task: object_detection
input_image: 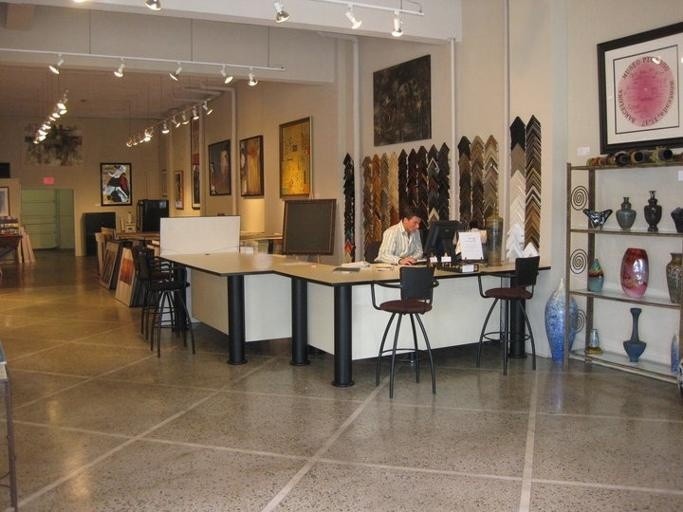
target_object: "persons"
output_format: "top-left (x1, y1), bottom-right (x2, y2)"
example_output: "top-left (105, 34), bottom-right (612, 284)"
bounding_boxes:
top-left (374, 207), bottom-right (423, 266)
top-left (240, 151), bottom-right (247, 196)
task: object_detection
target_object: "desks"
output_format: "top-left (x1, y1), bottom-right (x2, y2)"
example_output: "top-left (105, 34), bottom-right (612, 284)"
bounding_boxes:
top-left (160, 250), bottom-right (281, 365)
top-left (280, 262), bottom-right (550, 392)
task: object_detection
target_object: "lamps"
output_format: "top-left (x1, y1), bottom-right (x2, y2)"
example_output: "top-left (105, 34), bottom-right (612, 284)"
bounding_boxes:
top-left (32, 87), bottom-right (70, 145)
top-left (47, 51), bottom-right (258, 88)
top-left (144, 1), bottom-right (428, 39)
top-left (125, 101), bottom-right (212, 149)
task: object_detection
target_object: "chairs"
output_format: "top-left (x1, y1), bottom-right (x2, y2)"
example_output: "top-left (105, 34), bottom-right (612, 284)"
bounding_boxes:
top-left (368, 265), bottom-right (440, 399)
top-left (131, 246), bottom-right (180, 341)
top-left (478, 256), bottom-right (541, 375)
top-left (137, 250), bottom-right (196, 359)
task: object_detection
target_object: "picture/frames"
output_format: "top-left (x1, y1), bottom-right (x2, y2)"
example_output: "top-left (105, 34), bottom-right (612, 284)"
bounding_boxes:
top-left (278, 114), bottom-right (313, 199)
top-left (207, 139), bottom-right (232, 196)
top-left (0, 184), bottom-right (13, 219)
top-left (239, 135), bottom-right (264, 197)
top-left (96, 239), bottom-right (122, 291)
top-left (595, 18), bottom-right (682, 155)
top-left (114, 246), bottom-right (135, 307)
top-left (160, 115), bottom-right (200, 209)
top-left (100, 162), bottom-right (133, 206)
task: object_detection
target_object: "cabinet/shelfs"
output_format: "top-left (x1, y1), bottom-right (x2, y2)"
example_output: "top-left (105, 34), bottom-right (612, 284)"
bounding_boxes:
top-left (562, 159), bottom-right (683, 388)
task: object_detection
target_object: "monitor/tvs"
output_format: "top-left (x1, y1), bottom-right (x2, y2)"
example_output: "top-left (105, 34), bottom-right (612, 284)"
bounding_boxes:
top-left (423, 220), bottom-right (461, 264)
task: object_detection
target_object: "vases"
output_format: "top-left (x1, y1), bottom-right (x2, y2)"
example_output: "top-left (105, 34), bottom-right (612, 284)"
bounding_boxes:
top-left (665, 249), bottom-right (682, 303)
top-left (616, 246), bottom-right (648, 298)
top-left (669, 203), bottom-right (683, 233)
top-left (670, 328), bottom-right (678, 376)
top-left (542, 280), bottom-right (577, 367)
top-left (621, 304), bottom-right (646, 364)
top-left (614, 198), bottom-right (636, 231)
top-left (586, 257), bottom-right (605, 294)
top-left (641, 189), bottom-right (662, 232)
top-left (589, 329), bottom-right (599, 348)
